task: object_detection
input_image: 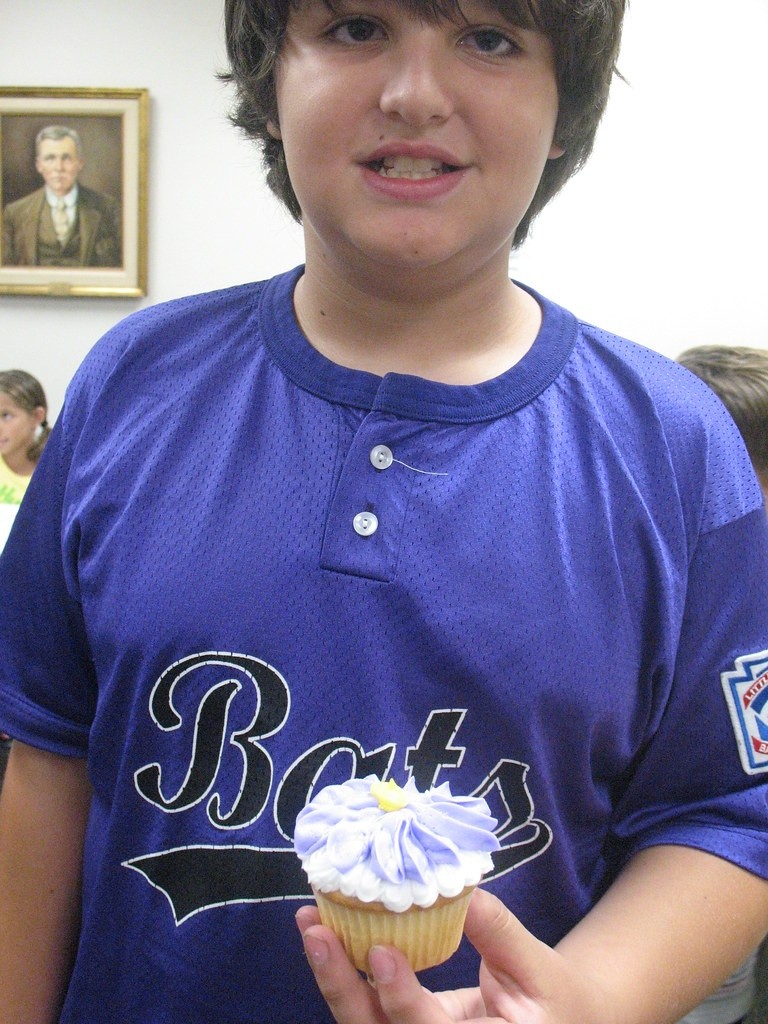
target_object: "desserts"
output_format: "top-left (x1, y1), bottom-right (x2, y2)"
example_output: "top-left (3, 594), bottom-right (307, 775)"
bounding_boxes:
top-left (294, 774), bottom-right (502, 971)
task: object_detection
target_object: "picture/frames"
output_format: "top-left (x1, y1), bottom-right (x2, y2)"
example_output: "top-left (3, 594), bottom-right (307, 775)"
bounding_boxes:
top-left (0, 85), bottom-right (150, 299)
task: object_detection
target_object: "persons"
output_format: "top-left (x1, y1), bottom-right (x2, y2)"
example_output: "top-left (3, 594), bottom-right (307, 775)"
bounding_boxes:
top-left (0, 0), bottom-right (768, 1024)
top-left (674, 346), bottom-right (768, 1024)
top-left (0, 370), bottom-right (52, 504)
